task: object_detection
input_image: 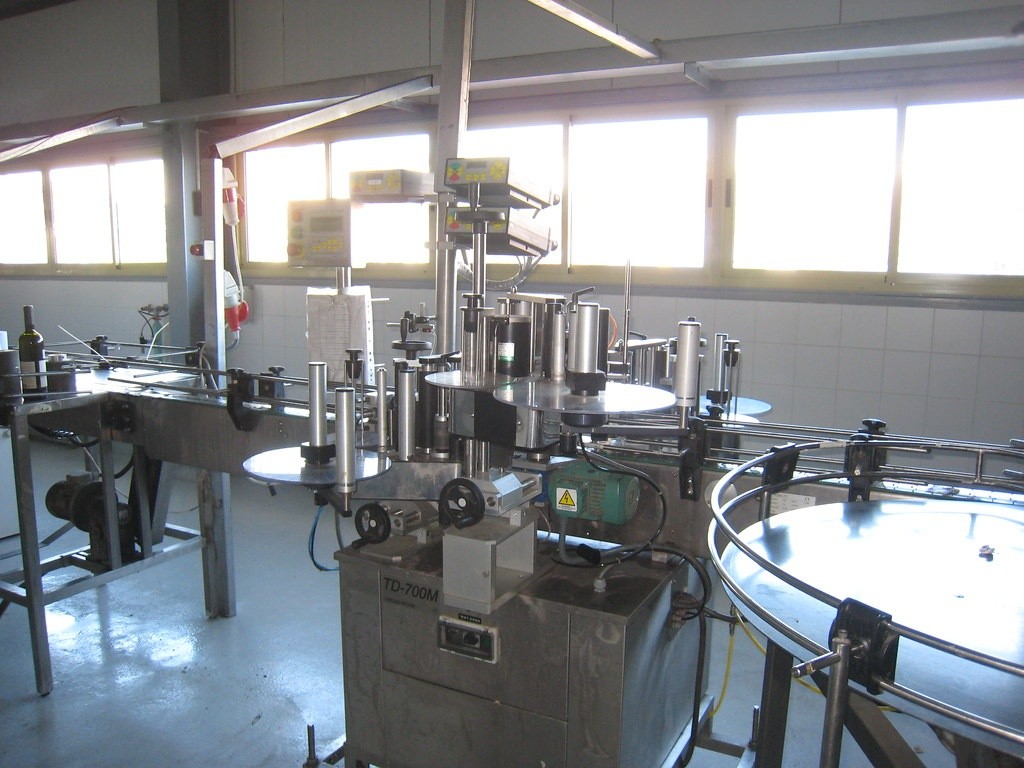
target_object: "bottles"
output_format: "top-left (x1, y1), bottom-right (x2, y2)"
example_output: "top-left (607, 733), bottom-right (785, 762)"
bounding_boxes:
top-left (19, 305), bottom-right (49, 399)
